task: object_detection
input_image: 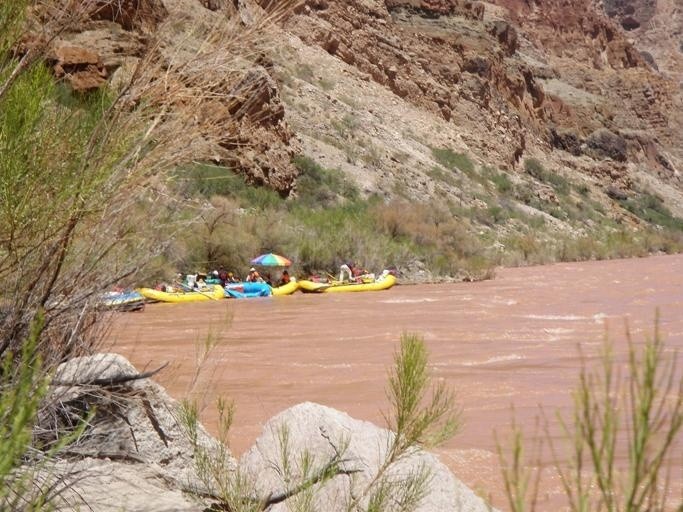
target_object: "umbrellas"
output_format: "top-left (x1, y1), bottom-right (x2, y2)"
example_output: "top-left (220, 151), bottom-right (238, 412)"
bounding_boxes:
top-left (248, 252), bottom-right (292, 268)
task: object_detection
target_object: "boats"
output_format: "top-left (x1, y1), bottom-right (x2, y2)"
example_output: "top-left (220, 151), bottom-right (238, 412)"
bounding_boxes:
top-left (139, 276), bottom-right (297, 304)
top-left (299, 272), bottom-right (396, 293)
top-left (50, 293), bottom-right (144, 312)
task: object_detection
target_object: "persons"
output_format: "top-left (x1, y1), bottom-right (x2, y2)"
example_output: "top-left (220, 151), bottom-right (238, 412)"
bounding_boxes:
top-left (110, 261), bottom-right (402, 297)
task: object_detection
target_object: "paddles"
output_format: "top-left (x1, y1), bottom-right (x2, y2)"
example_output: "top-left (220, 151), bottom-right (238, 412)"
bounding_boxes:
top-left (314, 275), bottom-right (360, 291)
